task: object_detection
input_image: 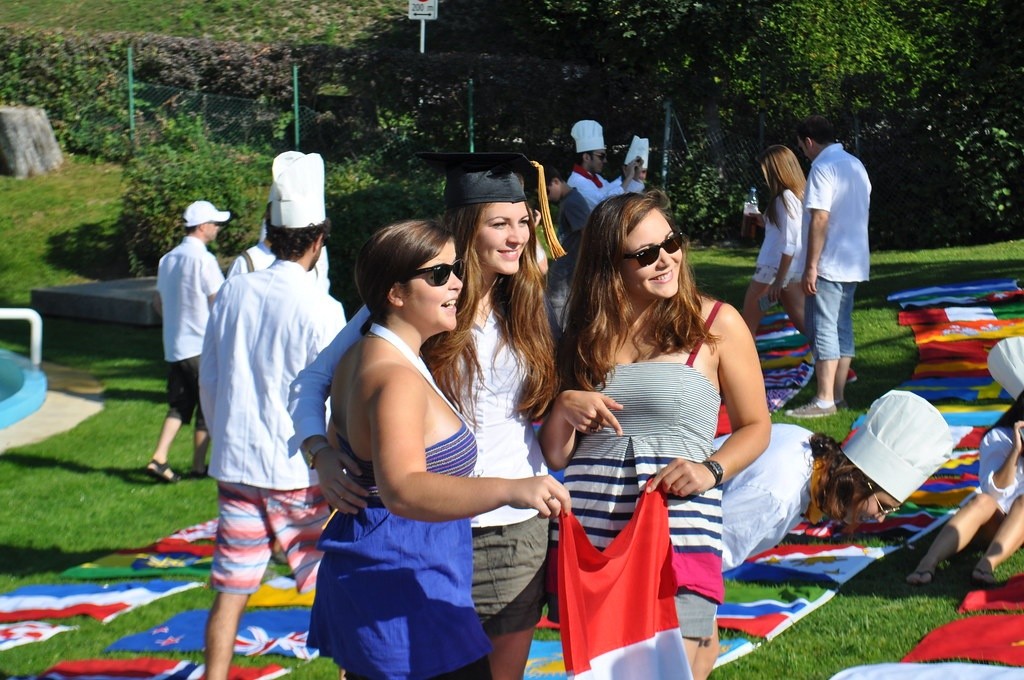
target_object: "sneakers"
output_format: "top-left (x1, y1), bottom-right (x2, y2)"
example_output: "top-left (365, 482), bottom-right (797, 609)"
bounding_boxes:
top-left (785, 400), bottom-right (837, 417)
top-left (836, 401), bottom-right (849, 410)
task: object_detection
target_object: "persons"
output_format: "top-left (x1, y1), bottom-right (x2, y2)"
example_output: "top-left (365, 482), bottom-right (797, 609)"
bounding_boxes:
top-left (784, 115), bottom-right (871, 418)
top-left (710, 389), bottom-right (954, 571)
top-left (286, 153), bottom-right (562, 680)
top-left (906, 337), bottom-right (1024, 582)
top-left (542, 159), bottom-right (591, 334)
top-left (566, 120), bottom-right (644, 211)
top-left (741, 144), bottom-right (857, 383)
top-left (302, 220), bottom-right (572, 680)
top-left (536, 191), bottom-right (772, 680)
top-left (199, 151), bottom-right (347, 680)
top-left (146, 201), bottom-right (231, 481)
top-left (611, 135), bottom-right (649, 185)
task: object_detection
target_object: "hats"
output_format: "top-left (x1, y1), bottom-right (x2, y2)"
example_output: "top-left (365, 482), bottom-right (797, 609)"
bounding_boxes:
top-left (625, 135), bottom-right (649, 169)
top-left (840, 390), bottom-right (953, 503)
top-left (987, 336), bottom-right (1024, 402)
top-left (271, 151), bottom-right (326, 227)
top-left (415, 152), bottom-right (569, 260)
top-left (570, 120), bottom-right (607, 152)
top-left (183, 201), bottom-right (231, 227)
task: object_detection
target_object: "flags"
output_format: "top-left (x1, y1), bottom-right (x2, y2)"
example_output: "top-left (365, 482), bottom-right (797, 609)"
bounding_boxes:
top-left (558, 478), bottom-right (696, 680)
top-left (0, 282), bottom-right (1024, 680)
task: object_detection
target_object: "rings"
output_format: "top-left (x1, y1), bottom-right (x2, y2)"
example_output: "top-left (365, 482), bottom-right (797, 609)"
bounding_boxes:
top-left (545, 496), bottom-right (555, 503)
top-left (591, 423), bottom-right (599, 432)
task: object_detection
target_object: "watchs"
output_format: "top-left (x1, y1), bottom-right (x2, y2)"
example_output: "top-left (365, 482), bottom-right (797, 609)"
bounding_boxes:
top-left (704, 461), bottom-right (723, 485)
top-left (302, 441), bottom-right (329, 469)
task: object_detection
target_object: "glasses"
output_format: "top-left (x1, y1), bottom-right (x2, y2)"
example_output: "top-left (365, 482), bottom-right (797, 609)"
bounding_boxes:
top-left (399, 259), bottom-right (464, 286)
top-left (624, 232), bottom-right (683, 267)
top-left (866, 479), bottom-right (900, 519)
top-left (589, 151), bottom-right (606, 161)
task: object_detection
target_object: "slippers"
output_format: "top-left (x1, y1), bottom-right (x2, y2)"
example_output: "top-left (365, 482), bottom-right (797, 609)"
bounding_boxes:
top-left (973, 569), bottom-right (996, 589)
top-left (906, 570), bottom-right (934, 587)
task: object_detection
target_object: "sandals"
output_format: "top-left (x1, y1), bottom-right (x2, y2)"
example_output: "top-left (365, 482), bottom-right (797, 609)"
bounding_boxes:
top-left (147, 459), bottom-right (182, 482)
top-left (190, 466), bottom-right (208, 480)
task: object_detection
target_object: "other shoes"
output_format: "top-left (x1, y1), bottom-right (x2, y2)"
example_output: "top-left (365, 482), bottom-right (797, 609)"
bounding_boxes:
top-left (847, 369), bottom-right (857, 383)
